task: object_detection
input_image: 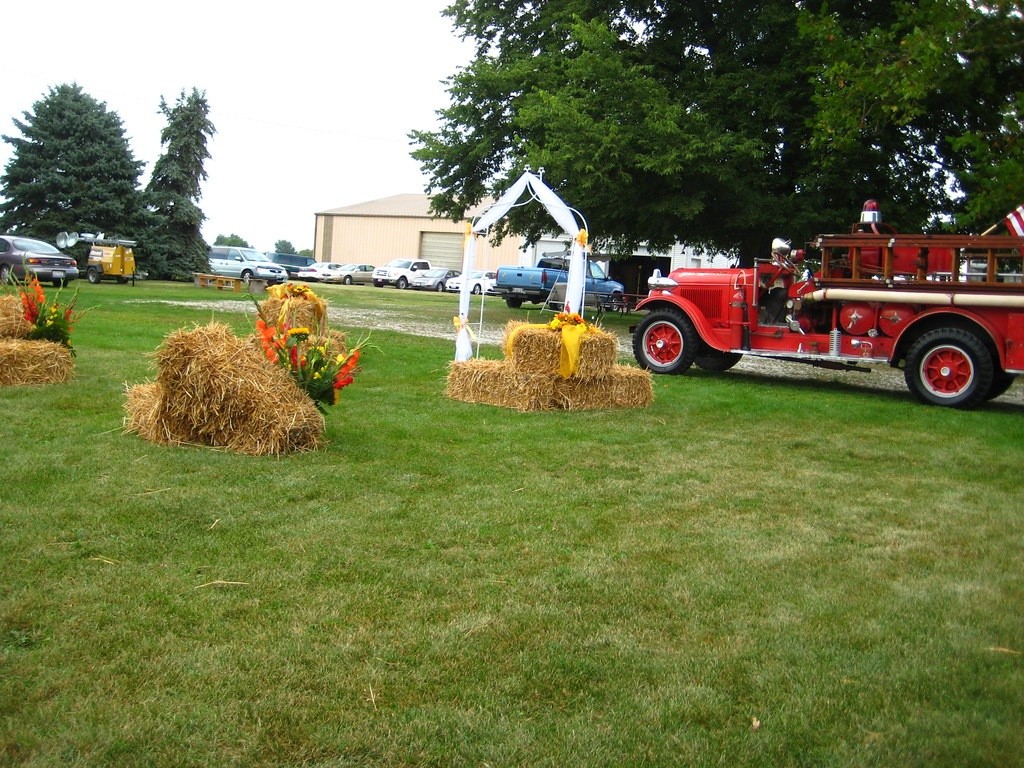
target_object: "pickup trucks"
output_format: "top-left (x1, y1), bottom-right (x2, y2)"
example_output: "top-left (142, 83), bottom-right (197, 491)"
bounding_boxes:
top-left (493, 258), bottom-right (624, 312)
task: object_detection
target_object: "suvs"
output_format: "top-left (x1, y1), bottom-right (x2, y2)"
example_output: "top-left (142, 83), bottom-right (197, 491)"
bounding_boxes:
top-left (372, 259), bottom-right (431, 289)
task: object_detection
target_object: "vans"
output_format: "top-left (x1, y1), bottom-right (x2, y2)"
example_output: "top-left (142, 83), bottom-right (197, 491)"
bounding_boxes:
top-left (273, 253), bottom-right (317, 280)
top-left (209, 246), bottom-right (288, 283)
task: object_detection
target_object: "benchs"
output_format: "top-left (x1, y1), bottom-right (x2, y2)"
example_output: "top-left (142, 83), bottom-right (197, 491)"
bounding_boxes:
top-left (194, 272), bottom-right (268, 294)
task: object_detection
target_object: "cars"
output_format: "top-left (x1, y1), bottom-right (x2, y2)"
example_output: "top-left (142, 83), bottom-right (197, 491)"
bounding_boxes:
top-left (320, 264), bottom-right (376, 285)
top-left (445, 271), bottom-right (497, 295)
top-left (0, 236), bottom-right (79, 288)
top-left (412, 268), bottom-right (461, 293)
top-left (297, 263), bottom-right (342, 282)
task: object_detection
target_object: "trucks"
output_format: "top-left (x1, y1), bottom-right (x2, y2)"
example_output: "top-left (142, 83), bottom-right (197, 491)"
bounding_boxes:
top-left (629, 199), bottom-right (1024, 407)
